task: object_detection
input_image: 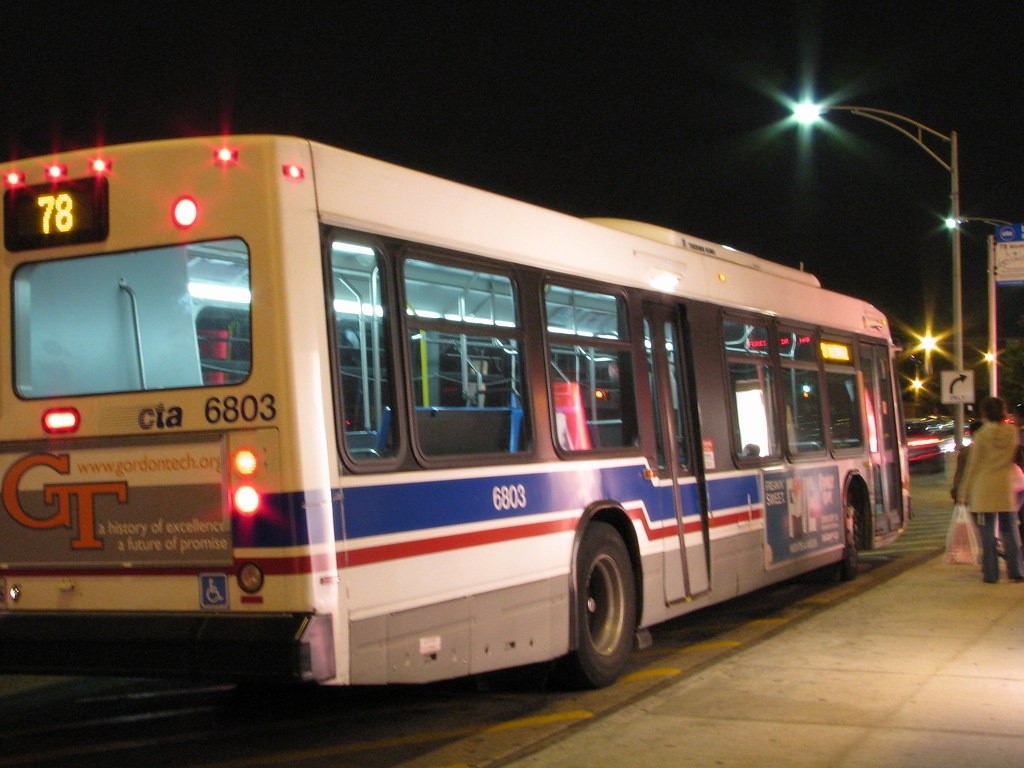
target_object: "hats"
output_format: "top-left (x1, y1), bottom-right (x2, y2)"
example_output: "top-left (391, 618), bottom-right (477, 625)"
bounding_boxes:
top-left (744, 444), bottom-right (760, 453)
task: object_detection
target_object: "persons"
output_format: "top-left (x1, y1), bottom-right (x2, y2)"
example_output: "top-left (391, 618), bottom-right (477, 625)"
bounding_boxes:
top-left (956, 395), bottom-right (1024, 583)
top-left (951, 406), bottom-right (1024, 573)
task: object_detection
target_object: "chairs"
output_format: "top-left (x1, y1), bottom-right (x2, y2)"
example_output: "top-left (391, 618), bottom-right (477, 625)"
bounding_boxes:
top-left (549, 382), bottom-right (593, 452)
top-left (787, 442), bottom-right (820, 454)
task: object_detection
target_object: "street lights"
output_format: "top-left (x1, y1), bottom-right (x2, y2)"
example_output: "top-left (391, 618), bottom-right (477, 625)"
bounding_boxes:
top-left (794, 99), bottom-right (964, 452)
top-left (941, 213), bottom-right (1012, 402)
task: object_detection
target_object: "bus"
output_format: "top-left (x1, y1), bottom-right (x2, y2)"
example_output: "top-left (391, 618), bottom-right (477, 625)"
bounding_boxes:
top-left (0, 133), bottom-right (912, 689)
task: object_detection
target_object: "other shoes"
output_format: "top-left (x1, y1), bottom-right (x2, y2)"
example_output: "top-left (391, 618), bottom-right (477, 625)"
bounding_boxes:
top-left (1015, 576), bottom-right (1024, 581)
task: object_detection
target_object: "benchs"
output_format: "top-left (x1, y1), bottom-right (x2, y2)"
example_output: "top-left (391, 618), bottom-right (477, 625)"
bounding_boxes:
top-left (347, 406), bottom-right (528, 462)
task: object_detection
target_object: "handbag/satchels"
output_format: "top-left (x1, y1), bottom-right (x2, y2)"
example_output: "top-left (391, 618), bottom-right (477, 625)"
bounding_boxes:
top-left (943, 505), bottom-right (979, 565)
